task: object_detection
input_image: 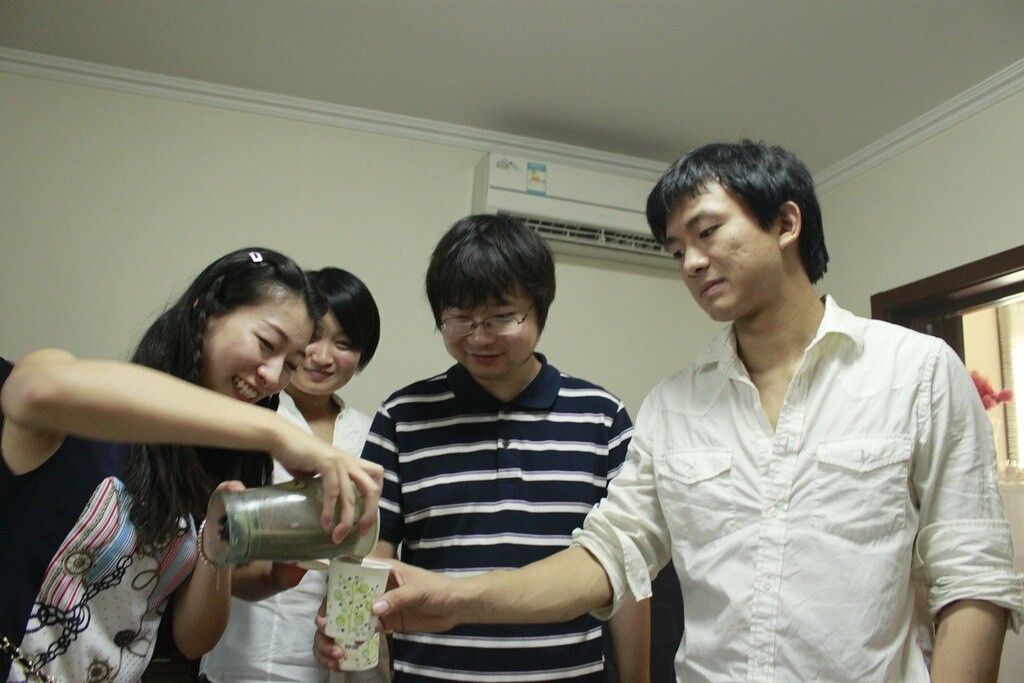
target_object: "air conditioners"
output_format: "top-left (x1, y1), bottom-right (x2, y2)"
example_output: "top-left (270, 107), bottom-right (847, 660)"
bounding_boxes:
top-left (469, 152), bottom-right (681, 273)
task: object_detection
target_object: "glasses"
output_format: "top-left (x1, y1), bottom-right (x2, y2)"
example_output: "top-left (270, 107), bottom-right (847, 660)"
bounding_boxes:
top-left (434, 301), bottom-right (536, 337)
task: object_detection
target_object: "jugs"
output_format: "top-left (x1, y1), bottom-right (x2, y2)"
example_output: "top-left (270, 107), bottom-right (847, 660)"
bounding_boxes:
top-left (207, 476), bottom-right (380, 563)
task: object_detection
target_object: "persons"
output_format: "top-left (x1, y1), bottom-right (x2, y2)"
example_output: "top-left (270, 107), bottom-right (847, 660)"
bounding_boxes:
top-left (0, 247), bottom-right (384, 683)
top-left (315, 214), bottom-right (652, 683)
top-left (321, 141), bottom-right (1022, 683)
top-left (198, 265), bottom-right (381, 683)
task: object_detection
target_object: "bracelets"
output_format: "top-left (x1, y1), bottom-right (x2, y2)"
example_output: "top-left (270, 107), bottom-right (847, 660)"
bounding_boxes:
top-left (198, 517), bottom-right (231, 572)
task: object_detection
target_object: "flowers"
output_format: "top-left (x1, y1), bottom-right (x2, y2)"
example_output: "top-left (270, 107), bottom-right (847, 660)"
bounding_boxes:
top-left (968, 371), bottom-right (1013, 409)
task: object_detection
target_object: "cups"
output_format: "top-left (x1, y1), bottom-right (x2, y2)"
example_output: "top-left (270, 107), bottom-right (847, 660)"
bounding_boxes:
top-left (325, 558), bottom-right (392, 641)
top-left (332, 632), bottom-right (380, 672)
